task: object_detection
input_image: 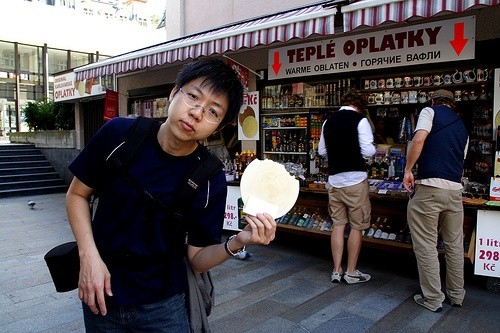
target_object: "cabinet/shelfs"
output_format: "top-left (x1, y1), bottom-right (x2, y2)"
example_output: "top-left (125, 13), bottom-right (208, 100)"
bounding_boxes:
top-left (258, 64), bottom-right (491, 254)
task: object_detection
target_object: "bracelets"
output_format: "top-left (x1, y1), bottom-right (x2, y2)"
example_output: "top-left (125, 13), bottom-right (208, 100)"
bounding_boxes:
top-left (225, 235), bottom-right (245, 257)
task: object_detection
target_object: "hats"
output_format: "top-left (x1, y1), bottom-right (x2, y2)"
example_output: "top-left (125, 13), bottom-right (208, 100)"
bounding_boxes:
top-left (425, 89), bottom-right (454, 107)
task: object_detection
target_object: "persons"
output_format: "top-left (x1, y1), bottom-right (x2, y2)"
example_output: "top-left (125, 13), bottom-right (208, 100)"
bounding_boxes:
top-left (403, 89), bottom-right (469, 312)
top-left (318, 89), bottom-right (377, 285)
top-left (65, 58), bottom-right (277, 333)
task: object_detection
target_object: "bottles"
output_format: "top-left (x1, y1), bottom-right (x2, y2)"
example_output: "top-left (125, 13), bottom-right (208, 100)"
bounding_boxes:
top-left (370, 157), bottom-right (402, 177)
top-left (262, 78), bottom-right (351, 183)
top-left (235, 150), bottom-right (256, 173)
top-left (274, 205), bottom-right (470, 253)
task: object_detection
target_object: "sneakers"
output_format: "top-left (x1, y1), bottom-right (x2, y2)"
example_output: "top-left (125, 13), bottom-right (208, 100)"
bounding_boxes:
top-left (343, 270), bottom-right (372, 284)
top-left (451, 301), bottom-right (462, 307)
top-left (414, 294), bottom-right (443, 312)
top-left (331, 272), bottom-right (344, 282)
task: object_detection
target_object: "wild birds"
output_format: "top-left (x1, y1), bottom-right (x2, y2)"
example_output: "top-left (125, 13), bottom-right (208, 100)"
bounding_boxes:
top-left (27, 200), bottom-right (36, 209)
top-left (238, 250), bottom-right (254, 260)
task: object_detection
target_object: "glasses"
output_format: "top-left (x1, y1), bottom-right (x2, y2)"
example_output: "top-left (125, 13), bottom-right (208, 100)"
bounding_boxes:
top-left (180, 88), bottom-right (223, 124)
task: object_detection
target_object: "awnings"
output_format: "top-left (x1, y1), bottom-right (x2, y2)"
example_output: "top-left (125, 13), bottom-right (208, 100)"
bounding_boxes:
top-left (340, 0), bottom-right (500, 32)
top-left (74, 0), bottom-right (337, 82)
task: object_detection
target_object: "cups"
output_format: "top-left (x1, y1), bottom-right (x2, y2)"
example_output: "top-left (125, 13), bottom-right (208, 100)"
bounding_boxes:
top-left (365, 69), bottom-right (488, 105)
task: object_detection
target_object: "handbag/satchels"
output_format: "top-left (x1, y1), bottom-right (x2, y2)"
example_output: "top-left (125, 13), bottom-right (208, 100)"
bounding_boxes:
top-left (44, 242), bottom-right (81, 293)
top-left (196, 270), bottom-right (215, 316)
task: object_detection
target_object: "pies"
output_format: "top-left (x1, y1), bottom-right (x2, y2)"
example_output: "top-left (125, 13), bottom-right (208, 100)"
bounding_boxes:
top-left (240, 158), bottom-right (299, 219)
